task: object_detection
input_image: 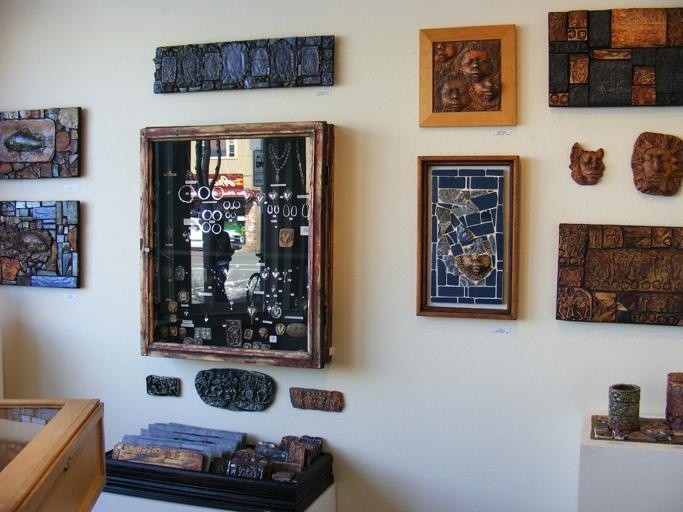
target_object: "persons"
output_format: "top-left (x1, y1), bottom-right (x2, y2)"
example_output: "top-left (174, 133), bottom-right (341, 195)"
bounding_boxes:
top-left (578, 148), bottom-right (604, 185)
top-left (434, 41), bottom-right (499, 113)
top-left (641, 146), bottom-right (673, 186)
top-left (456, 252), bottom-right (494, 280)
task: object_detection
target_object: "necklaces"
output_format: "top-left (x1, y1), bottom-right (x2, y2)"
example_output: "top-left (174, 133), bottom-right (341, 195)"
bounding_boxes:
top-left (295, 135), bottom-right (305, 184)
top-left (266, 143), bottom-right (292, 184)
top-left (191, 257), bottom-right (307, 325)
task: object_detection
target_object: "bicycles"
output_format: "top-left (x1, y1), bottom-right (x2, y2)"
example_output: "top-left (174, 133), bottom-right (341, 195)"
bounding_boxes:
top-left (245, 252), bottom-right (265, 305)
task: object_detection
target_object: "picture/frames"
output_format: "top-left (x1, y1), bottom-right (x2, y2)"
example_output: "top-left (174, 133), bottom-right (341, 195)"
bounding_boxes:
top-left (418, 24), bottom-right (518, 128)
top-left (140, 121), bottom-right (335, 369)
top-left (415, 155), bottom-right (520, 320)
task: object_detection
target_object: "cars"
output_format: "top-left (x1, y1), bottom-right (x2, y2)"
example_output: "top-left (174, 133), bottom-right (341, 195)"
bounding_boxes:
top-left (184, 217), bottom-right (242, 253)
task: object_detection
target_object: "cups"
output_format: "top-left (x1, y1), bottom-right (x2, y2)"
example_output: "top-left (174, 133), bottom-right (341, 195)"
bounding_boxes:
top-left (667, 372), bottom-right (682, 432)
